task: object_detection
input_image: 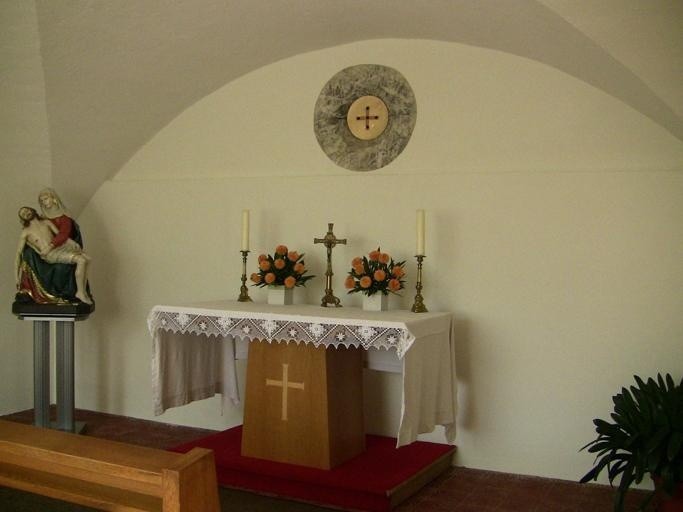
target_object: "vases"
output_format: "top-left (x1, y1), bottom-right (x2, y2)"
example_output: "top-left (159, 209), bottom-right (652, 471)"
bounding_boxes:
top-left (362, 290), bottom-right (388, 311)
top-left (268, 289), bottom-right (293, 305)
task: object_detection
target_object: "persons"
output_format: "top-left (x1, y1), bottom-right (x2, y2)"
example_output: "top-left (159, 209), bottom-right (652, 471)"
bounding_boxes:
top-left (14, 207), bottom-right (93, 306)
top-left (15, 188), bottom-right (90, 305)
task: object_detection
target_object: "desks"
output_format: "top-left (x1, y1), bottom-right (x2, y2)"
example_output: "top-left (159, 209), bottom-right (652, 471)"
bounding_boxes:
top-left (148, 300), bottom-right (457, 511)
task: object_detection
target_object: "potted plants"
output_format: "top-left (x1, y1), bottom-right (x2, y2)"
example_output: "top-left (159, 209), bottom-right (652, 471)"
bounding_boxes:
top-left (580, 372), bottom-right (683, 512)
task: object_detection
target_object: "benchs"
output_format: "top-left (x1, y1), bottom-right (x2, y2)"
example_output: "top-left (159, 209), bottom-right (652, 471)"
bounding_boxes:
top-left (0, 419), bottom-right (221, 512)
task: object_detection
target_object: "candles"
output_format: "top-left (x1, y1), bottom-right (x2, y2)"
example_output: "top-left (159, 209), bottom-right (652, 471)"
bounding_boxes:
top-left (242, 210), bottom-right (249, 251)
top-left (417, 209), bottom-right (425, 255)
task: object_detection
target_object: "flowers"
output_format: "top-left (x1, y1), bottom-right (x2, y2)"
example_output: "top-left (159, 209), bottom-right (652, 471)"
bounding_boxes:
top-left (249, 246), bottom-right (315, 289)
top-left (345, 246), bottom-right (406, 297)
